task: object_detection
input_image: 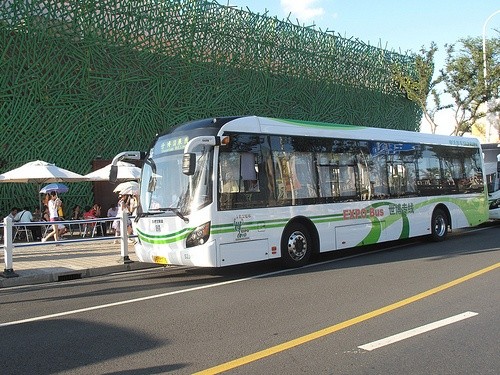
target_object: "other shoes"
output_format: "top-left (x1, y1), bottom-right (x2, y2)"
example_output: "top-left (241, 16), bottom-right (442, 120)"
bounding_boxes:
top-left (41, 238), bottom-right (46, 242)
top-left (128, 239), bottom-right (133, 243)
top-left (114, 239), bottom-right (119, 244)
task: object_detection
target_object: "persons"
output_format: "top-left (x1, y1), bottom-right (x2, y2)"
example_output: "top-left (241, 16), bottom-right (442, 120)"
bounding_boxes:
top-left (83, 191), bottom-right (139, 244)
top-left (33, 207), bottom-right (46, 222)
top-left (58, 204), bottom-right (64, 217)
top-left (13, 207), bottom-right (38, 242)
top-left (70, 205), bottom-right (86, 229)
top-left (42, 191), bottom-right (65, 247)
top-left (41, 193), bottom-right (51, 221)
top-left (6, 207), bottom-right (18, 235)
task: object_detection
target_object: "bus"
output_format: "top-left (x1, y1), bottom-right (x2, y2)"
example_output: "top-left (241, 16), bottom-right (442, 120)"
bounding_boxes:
top-left (109, 116), bottom-right (500, 268)
top-left (480, 144), bottom-right (499, 217)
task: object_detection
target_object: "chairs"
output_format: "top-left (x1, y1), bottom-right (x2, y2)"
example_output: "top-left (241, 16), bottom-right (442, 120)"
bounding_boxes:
top-left (12, 224), bottom-right (33, 243)
top-left (83, 216), bottom-right (104, 237)
top-left (69, 223), bottom-right (84, 237)
top-left (42, 218), bottom-right (60, 242)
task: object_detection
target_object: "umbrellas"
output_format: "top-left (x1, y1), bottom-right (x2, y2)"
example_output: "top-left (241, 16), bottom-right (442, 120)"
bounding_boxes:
top-left (85, 158), bottom-right (164, 182)
top-left (112, 182), bottom-right (141, 192)
top-left (0, 159), bottom-right (85, 213)
top-left (38, 183), bottom-right (70, 194)
top-left (118, 186), bottom-right (142, 197)
